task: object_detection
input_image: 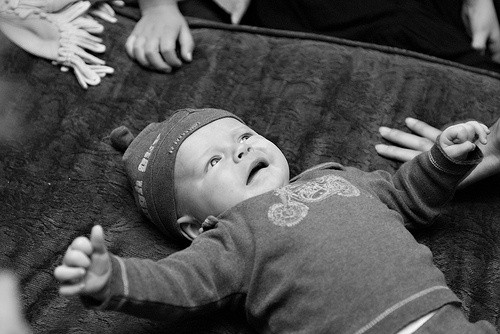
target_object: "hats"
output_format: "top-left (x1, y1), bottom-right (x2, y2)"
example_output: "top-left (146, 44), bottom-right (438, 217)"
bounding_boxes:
top-left (109, 107), bottom-right (245, 242)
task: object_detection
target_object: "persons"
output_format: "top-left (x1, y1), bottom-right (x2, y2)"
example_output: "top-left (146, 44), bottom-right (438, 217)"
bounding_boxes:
top-left (127, 0), bottom-right (500, 72)
top-left (55, 108), bottom-right (497, 334)
top-left (376, 117), bottom-right (500, 184)
